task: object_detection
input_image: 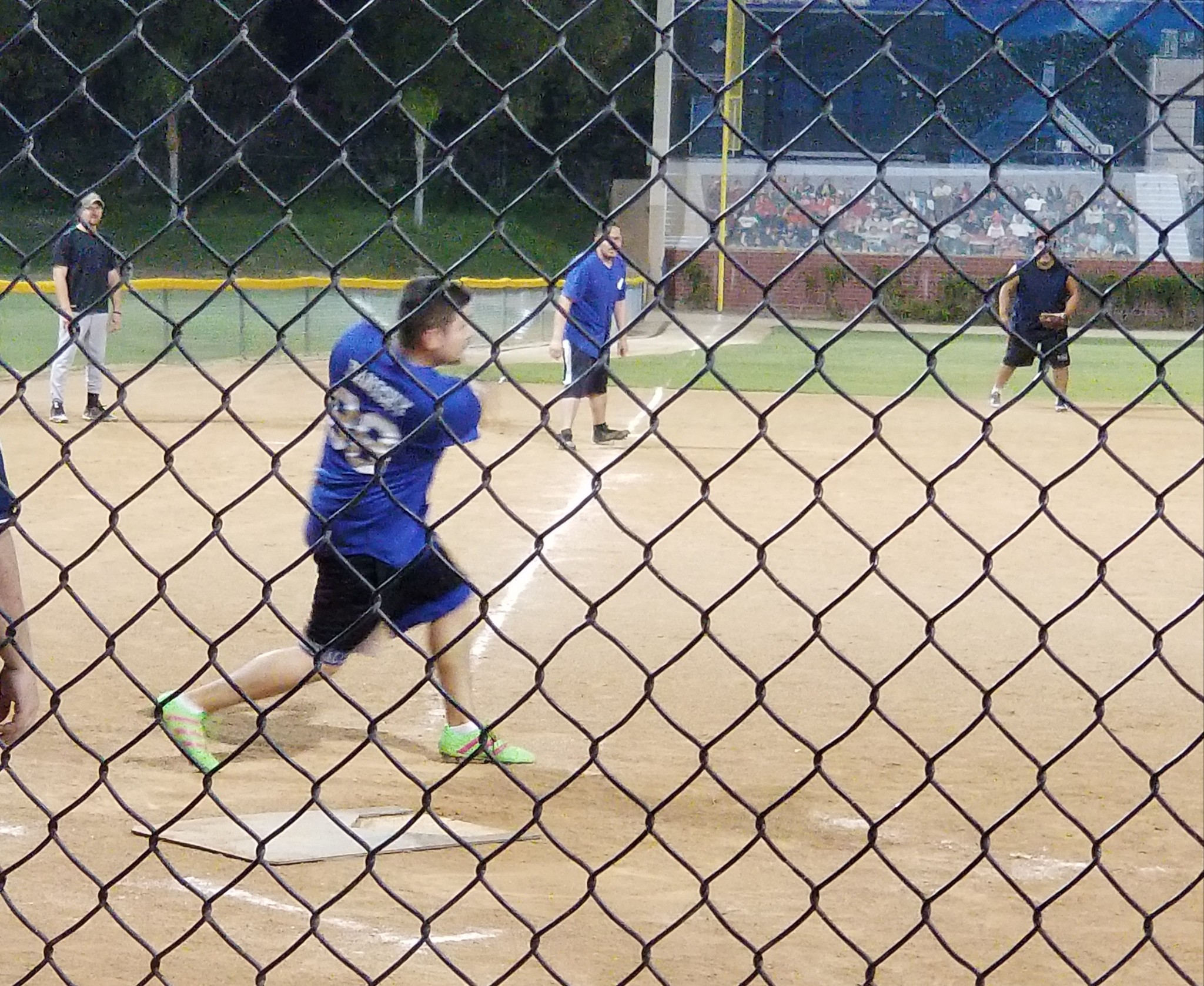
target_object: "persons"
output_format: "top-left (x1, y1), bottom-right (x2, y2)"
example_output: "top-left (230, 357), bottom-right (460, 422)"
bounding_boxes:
top-left (989, 235), bottom-right (1081, 411)
top-left (0, 454), bottom-right (41, 749)
top-left (159, 274), bottom-right (536, 774)
top-left (48, 192), bottom-right (122, 423)
top-left (706, 177), bottom-right (1137, 259)
top-left (550, 221), bottom-right (631, 451)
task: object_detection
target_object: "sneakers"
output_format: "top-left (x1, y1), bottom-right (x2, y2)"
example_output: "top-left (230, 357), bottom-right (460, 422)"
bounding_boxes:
top-left (554, 429), bottom-right (577, 451)
top-left (991, 391), bottom-right (1001, 406)
top-left (1055, 397), bottom-right (1068, 411)
top-left (83, 399), bottom-right (119, 422)
top-left (149, 691), bottom-right (221, 773)
top-left (592, 425), bottom-right (631, 444)
top-left (49, 402), bottom-right (69, 423)
top-left (439, 725), bottom-right (535, 765)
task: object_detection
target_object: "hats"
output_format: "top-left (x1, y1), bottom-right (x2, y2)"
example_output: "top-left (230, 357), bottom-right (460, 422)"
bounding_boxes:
top-left (81, 192), bottom-right (105, 210)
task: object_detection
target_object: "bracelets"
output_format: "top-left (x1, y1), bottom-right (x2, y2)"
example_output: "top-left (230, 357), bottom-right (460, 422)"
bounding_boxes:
top-left (113, 311), bottom-right (121, 314)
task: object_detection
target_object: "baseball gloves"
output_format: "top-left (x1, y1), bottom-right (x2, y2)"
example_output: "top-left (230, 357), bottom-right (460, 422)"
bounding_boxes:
top-left (1039, 313), bottom-right (1069, 331)
